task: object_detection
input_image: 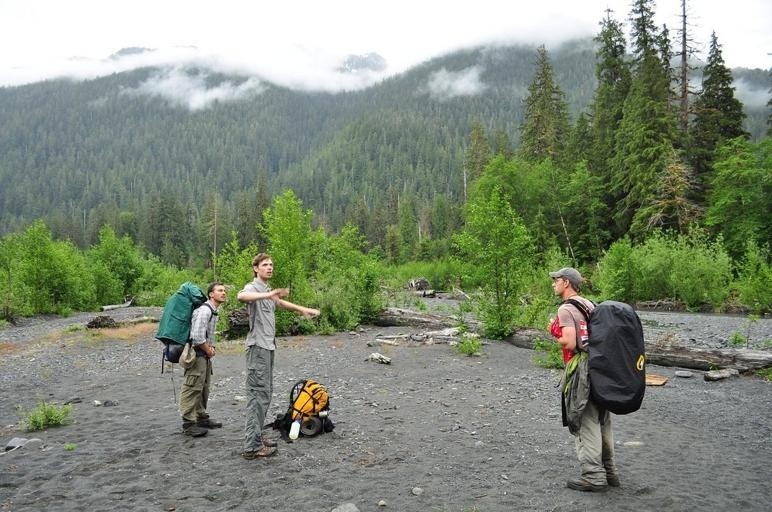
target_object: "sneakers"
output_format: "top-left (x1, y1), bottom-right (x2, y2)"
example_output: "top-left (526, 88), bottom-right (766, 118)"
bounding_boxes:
top-left (199, 420), bottom-right (222, 429)
top-left (262, 441), bottom-right (278, 447)
top-left (245, 445), bottom-right (277, 459)
top-left (184, 425), bottom-right (208, 437)
top-left (566, 475), bottom-right (620, 493)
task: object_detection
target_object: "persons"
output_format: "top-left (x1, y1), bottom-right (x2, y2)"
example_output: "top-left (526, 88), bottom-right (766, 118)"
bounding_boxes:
top-left (548, 267), bottom-right (621, 494)
top-left (178, 283), bottom-right (227, 438)
top-left (236, 253), bottom-right (321, 459)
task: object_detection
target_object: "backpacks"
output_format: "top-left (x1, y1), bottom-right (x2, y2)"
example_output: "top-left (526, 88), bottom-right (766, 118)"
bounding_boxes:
top-left (276, 379), bottom-right (329, 436)
top-left (155, 281), bottom-right (208, 363)
top-left (567, 296), bottom-right (645, 415)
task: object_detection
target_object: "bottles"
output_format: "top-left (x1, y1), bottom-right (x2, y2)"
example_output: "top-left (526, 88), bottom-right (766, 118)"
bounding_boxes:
top-left (289, 420), bottom-right (301, 440)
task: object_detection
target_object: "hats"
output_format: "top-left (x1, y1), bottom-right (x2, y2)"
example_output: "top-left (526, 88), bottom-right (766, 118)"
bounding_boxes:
top-left (549, 267), bottom-right (582, 285)
top-left (179, 344), bottom-right (197, 369)
